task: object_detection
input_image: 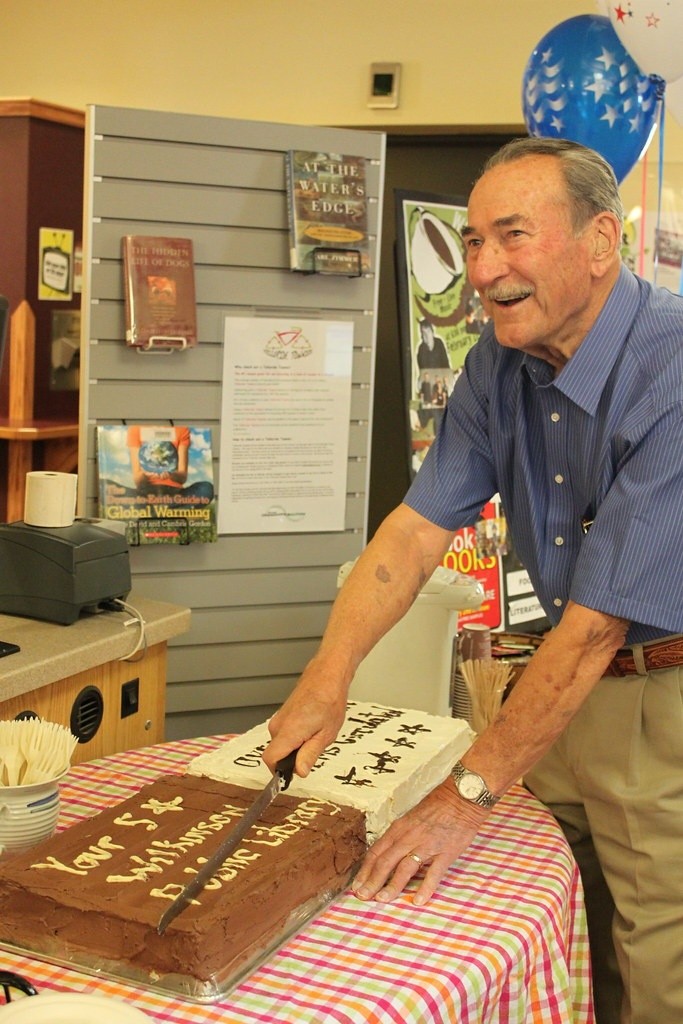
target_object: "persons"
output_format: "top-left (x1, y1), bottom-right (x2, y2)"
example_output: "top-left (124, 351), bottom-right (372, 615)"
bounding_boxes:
top-left (419, 373), bottom-right (449, 410)
top-left (416, 319), bottom-right (451, 370)
top-left (466, 297), bottom-right (487, 333)
top-left (478, 525), bottom-right (504, 555)
top-left (263, 131), bottom-right (683, 1024)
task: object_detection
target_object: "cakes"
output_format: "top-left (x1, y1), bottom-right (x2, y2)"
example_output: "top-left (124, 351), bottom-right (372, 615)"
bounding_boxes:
top-left (0, 773), bottom-right (366, 979)
top-left (187, 699), bottom-right (475, 845)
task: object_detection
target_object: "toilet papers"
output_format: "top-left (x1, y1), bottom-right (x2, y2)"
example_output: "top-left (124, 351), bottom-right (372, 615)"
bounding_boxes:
top-left (23, 470), bottom-right (79, 528)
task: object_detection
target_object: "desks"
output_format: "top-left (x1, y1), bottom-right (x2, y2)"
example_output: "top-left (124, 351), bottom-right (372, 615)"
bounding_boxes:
top-left (0, 732), bottom-right (599, 1024)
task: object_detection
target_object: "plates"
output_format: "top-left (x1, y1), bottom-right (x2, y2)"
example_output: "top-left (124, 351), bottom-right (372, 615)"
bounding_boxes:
top-left (453, 674), bottom-right (474, 722)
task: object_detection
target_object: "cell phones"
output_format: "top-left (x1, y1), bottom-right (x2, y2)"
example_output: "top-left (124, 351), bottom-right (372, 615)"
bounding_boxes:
top-left (0, 641), bottom-right (20, 658)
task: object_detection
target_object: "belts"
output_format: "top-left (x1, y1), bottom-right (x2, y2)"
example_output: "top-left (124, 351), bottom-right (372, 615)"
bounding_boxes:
top-left (605, 637), bottom-right (683, 675)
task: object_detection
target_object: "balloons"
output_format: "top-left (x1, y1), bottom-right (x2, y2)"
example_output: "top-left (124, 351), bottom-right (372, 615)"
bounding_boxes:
top-left (520, 0), bottom-right (683, 190)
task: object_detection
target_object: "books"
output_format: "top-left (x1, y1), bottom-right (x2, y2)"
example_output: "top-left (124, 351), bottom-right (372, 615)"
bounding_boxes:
top-left (282, 144), bottom-right (367, 276)
top-left (218, 312), bottom-right (348, 536)
top-left (96, 425), bottom-right (215, 548)
top-left (122, 234), bottom-right (196, 346)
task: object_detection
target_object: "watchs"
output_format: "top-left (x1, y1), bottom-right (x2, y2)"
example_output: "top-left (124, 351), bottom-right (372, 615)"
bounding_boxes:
top-left (449, 761), bottom-right (501, 811)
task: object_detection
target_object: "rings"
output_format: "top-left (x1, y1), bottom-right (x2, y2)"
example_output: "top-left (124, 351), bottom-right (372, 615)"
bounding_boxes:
top-left (407, 853), bottom-right (423, 869)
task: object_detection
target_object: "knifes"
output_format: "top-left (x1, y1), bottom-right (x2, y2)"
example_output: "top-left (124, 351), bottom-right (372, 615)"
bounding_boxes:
top-left (156, 751), bottom-right (298, 936)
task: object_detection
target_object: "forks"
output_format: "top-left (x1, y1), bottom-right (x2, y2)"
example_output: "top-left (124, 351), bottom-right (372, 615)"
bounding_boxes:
top-left (0, 716), bottom-right (79, 787)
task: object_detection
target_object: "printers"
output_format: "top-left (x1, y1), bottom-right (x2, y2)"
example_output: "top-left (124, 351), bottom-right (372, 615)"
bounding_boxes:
top-left (0, 516), bottom-right (132, 625)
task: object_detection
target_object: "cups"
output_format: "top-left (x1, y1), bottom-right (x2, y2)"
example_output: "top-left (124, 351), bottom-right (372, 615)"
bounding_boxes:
top-left (468, 687), bottom-right (506, 735)
top-left (1, 764), bottom-right (72, 854)
top-left (457, 621), bottom-right (491, 662)
